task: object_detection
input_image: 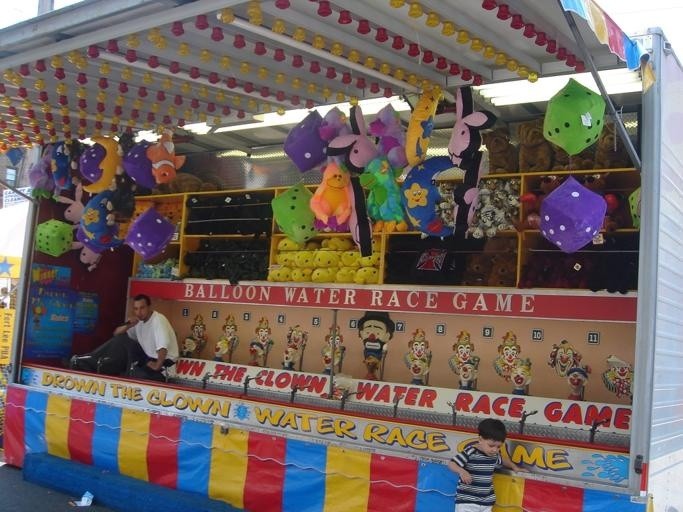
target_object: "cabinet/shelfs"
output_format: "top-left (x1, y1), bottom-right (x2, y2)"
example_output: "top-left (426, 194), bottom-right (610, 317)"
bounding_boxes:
top-left (116, 167), bottom-right (642, 289)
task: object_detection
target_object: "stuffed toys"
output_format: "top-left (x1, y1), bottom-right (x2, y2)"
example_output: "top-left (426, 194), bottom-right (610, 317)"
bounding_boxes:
top-left (31, 127), bottom-right (185, 281)
top-left (185, 193), bottom-right (276, 283)
top-left (25, 76), bottom-right (643, 287)
top-left (521, 77), bottom-right (642, 253)
top-left (270, 84), bottom-right (520, 288)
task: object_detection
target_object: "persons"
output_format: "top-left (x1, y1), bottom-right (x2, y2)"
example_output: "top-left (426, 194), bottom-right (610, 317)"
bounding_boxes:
top-left (69, 295), bottom-right (179, 381)
top-left (446, 418), bottom-right (531, 511)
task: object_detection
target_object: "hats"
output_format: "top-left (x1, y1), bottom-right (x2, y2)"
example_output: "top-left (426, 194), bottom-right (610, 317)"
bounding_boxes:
top-left (357, 311), bottom-right (395, 332)
